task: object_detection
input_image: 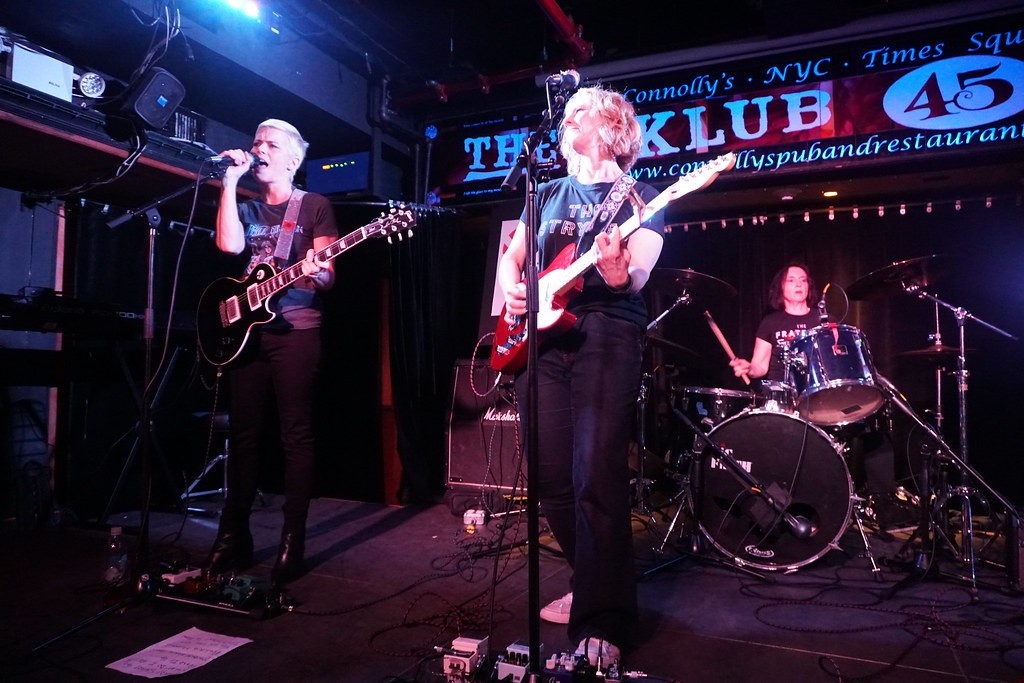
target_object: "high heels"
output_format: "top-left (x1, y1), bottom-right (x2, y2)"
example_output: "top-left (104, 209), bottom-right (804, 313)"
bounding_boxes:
top-left (199, 513), bottom-right (255, 575)
top-left (270, 503), bottom-right (307, 581)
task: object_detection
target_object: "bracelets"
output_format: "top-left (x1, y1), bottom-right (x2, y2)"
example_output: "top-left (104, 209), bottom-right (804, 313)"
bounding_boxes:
top-left (607, 274), bottom-right (633, 294)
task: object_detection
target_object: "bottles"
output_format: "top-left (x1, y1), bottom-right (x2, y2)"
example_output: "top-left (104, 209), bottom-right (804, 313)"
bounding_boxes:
top-left (102, 527), bottom-right (130, 613)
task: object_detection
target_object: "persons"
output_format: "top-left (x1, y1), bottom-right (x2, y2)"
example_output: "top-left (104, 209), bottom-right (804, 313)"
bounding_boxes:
top-left (501, 89), bottom-right (665, 670)
top-left (729, 263), bottom-right (923, 530)
top-left (204, 119), bottom-right (337, 581)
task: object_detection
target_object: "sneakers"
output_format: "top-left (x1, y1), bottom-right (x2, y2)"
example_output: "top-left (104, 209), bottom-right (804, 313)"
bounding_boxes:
top-left (576, 636), bottom-right (620, 669)
top-left (540, 590), bottom-right (572, 624)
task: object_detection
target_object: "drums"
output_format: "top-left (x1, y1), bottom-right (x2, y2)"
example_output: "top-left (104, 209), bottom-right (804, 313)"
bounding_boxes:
top-left (785, 325), bottom-right (888, 427)
top-left (686, 411), bottom-right (854, 572)
top-left (762, 379), bottom-right (797, 416)
top-left (672, 384), bottom-right (765, 476)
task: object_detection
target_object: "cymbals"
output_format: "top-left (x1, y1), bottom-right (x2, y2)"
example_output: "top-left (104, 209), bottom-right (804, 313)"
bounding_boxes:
top-left (650, 268), bottom-right (736, 296)
top-left (646, 335), bottom-right (707, 364)
top-left (883, 345), bottom-right (981, 363)
top-left (843, 255), bottom-right (953, 302)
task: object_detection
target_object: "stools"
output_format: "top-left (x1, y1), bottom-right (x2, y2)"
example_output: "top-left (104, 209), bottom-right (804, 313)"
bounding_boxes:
top-left (170, 411), bottom-right (270, 508)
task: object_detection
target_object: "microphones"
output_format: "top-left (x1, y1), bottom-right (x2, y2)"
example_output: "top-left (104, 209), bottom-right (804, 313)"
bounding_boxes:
top-left (204, 152), bottom-right (259, 168)
top-left (789, 514), bottom-right (811, 539)
top-left (545, 69), bottom-right (580, 88)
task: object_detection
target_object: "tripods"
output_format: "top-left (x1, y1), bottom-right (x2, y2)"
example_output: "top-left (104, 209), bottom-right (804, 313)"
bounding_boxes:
top-left (475, 527), bottom-right (566, 558)
top-left (877, 279), bottom-right (1024, 603)
top-left (27, 160), bottom-right (260, 654)
top-left (633, 371), bottom-right (791, 583)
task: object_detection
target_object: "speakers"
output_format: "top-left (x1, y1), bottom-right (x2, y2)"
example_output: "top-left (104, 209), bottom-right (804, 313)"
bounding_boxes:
top-left (443, 359), bottom-right (526, 496)
top-left (124, 67), bottom-right (187, 129)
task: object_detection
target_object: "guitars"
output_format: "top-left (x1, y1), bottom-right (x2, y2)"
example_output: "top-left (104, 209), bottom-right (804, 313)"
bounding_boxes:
top-left (490, 151), bottom-right (737, 376)
top-left (195, 203), bottom-right (418, 367)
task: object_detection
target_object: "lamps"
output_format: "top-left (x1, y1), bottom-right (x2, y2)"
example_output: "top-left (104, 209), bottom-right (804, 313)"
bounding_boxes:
top-left (80, 72), bottom-right (106, 97)
